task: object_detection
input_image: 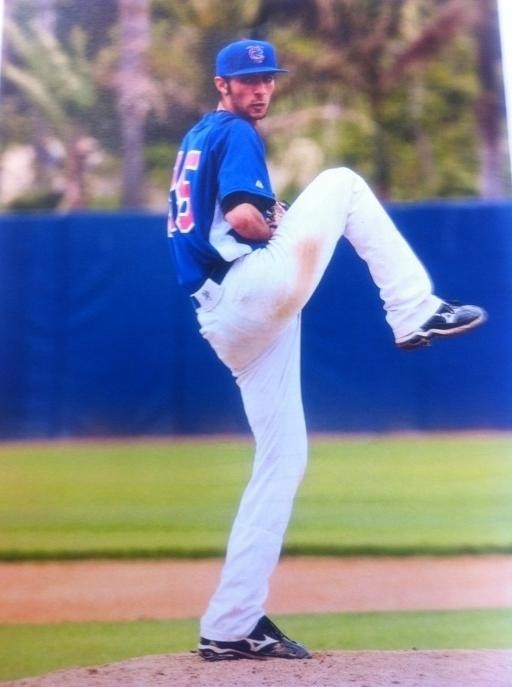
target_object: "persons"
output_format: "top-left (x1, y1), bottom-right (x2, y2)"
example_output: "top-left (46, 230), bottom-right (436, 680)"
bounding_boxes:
top-left (167, 39), bottom-right (489, 661)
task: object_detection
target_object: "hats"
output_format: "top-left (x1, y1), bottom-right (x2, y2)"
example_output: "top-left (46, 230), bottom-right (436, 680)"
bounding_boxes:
top-left (215, 38), bottom-right (289, 78)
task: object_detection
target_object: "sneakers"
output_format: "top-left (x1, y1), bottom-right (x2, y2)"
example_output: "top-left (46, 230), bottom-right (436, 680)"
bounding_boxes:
top-left (196, 615), bottom-right (314, 661)
top-left (392, 300), bottom-right (489, 348)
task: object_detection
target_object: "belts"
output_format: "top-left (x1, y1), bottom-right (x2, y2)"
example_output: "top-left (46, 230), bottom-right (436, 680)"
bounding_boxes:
top-left (189, 259), bottom-right (233, 312)
